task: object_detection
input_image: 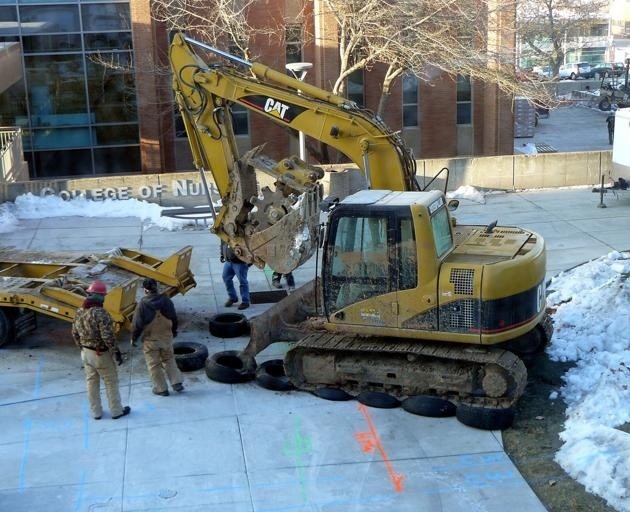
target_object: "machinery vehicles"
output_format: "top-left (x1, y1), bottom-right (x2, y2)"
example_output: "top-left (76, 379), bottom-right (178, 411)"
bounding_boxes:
top-left (0, 244), bottom-right (197, 347)
top-left (168, 33), bottom-right (554, 410)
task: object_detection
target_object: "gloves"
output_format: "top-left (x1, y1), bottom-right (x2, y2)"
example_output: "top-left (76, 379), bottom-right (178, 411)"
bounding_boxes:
top-left (131, 335), bottom-right (138, 346)
top-left (115, 350), bottom-right (122, 366)
top-left (173, 331), bottom-right (176, 337)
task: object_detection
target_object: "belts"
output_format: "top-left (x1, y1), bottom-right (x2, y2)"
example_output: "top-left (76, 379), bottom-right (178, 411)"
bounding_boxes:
top-left (81, 345), bottom-right (108, 352)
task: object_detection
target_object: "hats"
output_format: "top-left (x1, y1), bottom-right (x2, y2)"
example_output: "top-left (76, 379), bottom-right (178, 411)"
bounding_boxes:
top-left (141, 278), bottom-right (157, 288)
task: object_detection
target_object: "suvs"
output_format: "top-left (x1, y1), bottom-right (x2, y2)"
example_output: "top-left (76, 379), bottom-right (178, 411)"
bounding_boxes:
top-left (559, 62), bottom-right (591, 79)
top-left (589, 62), bottom-right (625, 78)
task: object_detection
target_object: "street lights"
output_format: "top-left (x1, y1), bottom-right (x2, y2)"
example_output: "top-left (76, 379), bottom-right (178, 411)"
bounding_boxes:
top-left (285, 62), bottom-right (313, 163)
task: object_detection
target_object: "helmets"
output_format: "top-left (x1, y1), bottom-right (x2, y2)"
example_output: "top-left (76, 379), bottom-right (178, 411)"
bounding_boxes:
top-left (86, 281), bottom-right (105, 293)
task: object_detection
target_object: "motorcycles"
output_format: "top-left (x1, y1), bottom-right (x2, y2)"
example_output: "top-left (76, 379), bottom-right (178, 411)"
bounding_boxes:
top-left (599, 82), bottom-right (630, 111)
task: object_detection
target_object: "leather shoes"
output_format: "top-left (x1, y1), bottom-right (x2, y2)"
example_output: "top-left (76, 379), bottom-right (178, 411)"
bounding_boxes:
top-left (271, 278), bottom-right (282, 289)
top-left (225, 298), bottom-right (238, 306)
top-left (95, 417), bottom-right (100, 419)
top-left (112, 406), bottom-right (130, 419)
top-left (173, 384), bottom-right (184, 391)
top-left (153, 388), bottom-right (169, 396)
top-left (288, 276), bottom-right (294, 286)
top-left (238, 303), bottom-right (249, 309)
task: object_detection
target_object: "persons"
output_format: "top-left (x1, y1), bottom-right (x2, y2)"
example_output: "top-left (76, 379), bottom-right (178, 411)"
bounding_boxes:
top-left (131, 278), bottom-right (185, 396)
top-left (605, 111), bottom-right (616, 145)
top-left (220, 237), bottom-right (253, 310)
top-left (72, 281), bottom-right (130, 420)
top-left (272, 270), bottom-right (296, 291)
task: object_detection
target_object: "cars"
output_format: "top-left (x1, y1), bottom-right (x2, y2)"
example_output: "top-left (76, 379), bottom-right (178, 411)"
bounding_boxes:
top-left (532, 66), bottom-right (553, 78)
